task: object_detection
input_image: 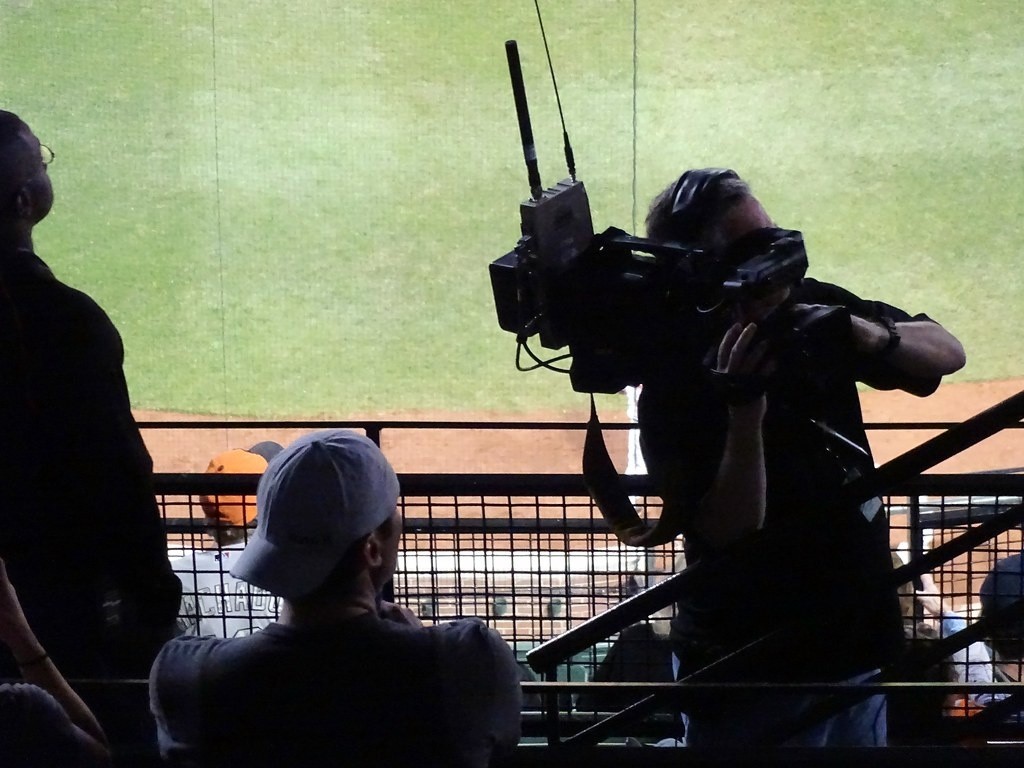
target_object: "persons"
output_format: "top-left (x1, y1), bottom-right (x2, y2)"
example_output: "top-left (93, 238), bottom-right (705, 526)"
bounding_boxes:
top-left (0, 108), bottom-right (183, 682)
top-left (636, 165), bottom-right (964, 748)
top-left (575, 552), bottom-right (679, 712)
top-left (0, 560), bottom-right (110, 768)
top-left (884, 543), bottom-right (1024, 746)
top-left (150, 429), bottom-right (523, 768)
top-left (169, 440), bottom-right (283, 640)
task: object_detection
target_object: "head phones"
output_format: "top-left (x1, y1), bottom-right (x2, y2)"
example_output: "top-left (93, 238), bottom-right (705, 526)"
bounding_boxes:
top-left (664, 166), bottom-right (741, 251)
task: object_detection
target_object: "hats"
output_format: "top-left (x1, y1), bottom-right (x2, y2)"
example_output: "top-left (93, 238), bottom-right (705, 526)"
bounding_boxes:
top-left (979, 553), bottom-right (1024, 657)
top-left (229, 430), bottom-right (403, 596)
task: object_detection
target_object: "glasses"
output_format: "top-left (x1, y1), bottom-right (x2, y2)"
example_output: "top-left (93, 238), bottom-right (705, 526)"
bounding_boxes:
top-left (14, 144), bottom-right (55, 197)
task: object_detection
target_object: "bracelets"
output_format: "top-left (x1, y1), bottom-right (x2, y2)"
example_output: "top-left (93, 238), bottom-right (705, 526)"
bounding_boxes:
top-left (875, 316), bottom-right (900, 357)
top-left (25, 654), bottom-right (52, 678)
